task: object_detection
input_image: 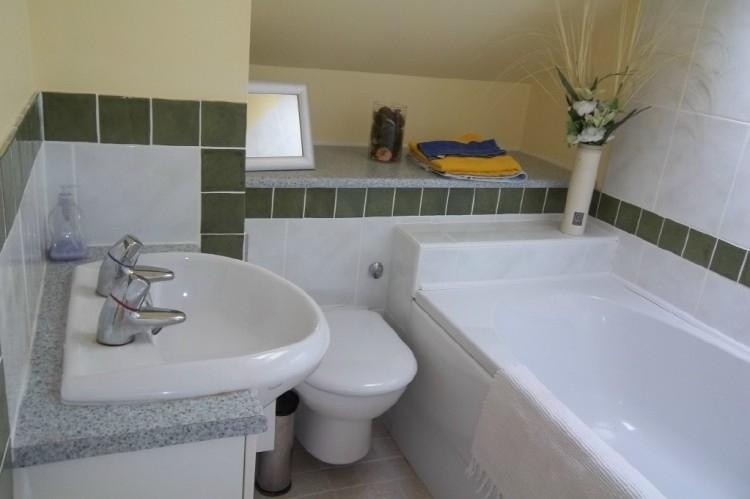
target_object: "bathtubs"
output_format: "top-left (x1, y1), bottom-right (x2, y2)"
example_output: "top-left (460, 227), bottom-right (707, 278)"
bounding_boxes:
top-left (415, 276), bottom-right (750, 499)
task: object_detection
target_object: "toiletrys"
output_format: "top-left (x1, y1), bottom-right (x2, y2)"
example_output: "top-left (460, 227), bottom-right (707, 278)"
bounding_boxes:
top-left (48, 182), bottom-right (88, 260)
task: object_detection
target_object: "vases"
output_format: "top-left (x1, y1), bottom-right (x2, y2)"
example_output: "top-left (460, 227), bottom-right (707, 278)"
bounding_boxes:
top-left (560, 142), bottom-right (603, 237)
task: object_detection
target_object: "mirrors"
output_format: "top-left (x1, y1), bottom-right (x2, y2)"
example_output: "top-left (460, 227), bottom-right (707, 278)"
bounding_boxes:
top-left (245, 81), bottom-right (317, 171)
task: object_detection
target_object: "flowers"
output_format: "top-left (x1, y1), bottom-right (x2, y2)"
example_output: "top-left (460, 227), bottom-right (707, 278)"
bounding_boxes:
top-left (477, 0), bottom-right (730, 148)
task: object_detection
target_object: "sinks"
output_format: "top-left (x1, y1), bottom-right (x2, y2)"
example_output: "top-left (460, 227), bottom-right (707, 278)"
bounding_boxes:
top-left (59, 250), bottom-right (331, 407)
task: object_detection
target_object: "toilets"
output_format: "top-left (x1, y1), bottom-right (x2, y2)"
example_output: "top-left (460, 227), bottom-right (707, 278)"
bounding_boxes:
top-left (296, 307), bottom-right (417, 465)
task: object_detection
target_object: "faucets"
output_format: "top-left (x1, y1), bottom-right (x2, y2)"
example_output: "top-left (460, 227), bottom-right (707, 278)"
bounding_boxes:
top-left (96, 234), bottom-right (174, 296)
top-left (96, 275), bottom-right (187, 345)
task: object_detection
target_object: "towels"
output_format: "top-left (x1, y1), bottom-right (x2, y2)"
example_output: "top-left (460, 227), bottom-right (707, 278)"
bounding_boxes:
top-left (407, 132), bottom-right (530, 176)
top-left (465, 360), bottom-right (665, 499)
